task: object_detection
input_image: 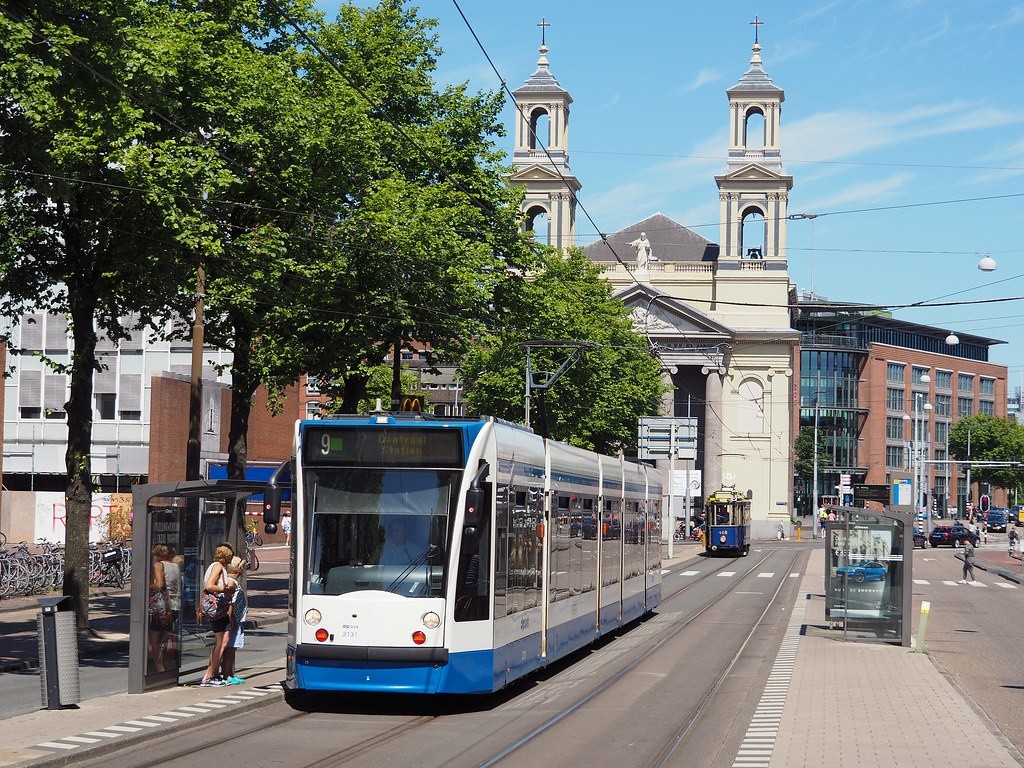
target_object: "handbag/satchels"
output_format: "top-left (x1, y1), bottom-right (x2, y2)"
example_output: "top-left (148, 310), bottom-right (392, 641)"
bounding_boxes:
top-left (201, 563), bottom-right (228, 620)
top-left (148, 562), bottom-right (173, 626)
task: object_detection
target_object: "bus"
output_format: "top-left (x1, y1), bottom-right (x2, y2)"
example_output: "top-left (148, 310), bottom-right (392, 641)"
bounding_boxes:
top-left (263, 340), bottom-right (664, 695)
top-left (700, 482), bottom-right (752, 558)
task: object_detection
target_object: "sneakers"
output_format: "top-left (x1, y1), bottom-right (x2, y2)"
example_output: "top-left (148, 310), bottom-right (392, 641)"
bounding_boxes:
top-left (200, 676), bottom-right (226, 687)
top-left (212, 674), bottom-right (231, 686)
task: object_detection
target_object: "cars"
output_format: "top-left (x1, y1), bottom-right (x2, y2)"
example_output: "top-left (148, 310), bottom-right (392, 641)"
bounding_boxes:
top-left (929, 524), bottom-right (981, 549)
top-left (973, 505), bottom-right (1024, 534)
top-left (835, 560), bottom-right (887, 584)
top-left (912, 526), bottom-right (928, 550)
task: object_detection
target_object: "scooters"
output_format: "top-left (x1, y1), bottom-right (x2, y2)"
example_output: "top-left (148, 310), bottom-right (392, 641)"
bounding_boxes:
top-left (672, 515), bottom-right (706, 542)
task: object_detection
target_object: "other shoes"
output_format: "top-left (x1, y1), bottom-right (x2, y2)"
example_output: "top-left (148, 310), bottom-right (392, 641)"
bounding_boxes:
top-left (959, 580), bottom-right (966, 584)
top-left (969, 581), bottom-right (976, 585)
top-left (222, 677), bottom-right (240, 684)
top-left (232, 676), bottom-right (246, 684)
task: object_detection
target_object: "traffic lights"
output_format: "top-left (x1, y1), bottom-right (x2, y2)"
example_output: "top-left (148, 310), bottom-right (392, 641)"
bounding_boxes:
top-left (980, 494), bottom-right (990, 513)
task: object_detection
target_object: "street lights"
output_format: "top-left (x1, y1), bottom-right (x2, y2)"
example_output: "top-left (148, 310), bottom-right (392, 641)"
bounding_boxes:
top-left (914, 392), bottom-right (923, 517)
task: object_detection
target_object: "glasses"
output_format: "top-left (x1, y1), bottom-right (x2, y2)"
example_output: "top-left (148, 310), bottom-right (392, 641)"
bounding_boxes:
top-left (391, 528), bottom-right (399, 533)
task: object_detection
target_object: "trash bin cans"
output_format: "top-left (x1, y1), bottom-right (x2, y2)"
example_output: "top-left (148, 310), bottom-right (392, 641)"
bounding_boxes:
top-left (36, 596), bottom-right (82, 712)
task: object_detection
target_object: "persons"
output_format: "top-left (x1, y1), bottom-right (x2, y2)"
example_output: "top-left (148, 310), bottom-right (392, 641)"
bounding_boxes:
top-left (351, 519), bottom-right (427, 566)
top-left (281, 511), bottom-right (291, 545)
top-left (151, 544), bottom-right (183, 673)
top-left (817, 505), bottom-right (835, 538)
top-left (777, 519), bottom-right (784, 540)
top-left (958, 537), bottom-right (976, 584)
top-left (947, 505), bottom-right (961, 533)
top-left (717, 507), bottom-right (729, 525)
top-left (198, 543), bottom-right (248, 687)
top-left (561, 498), bottom-right (660, 544)
top-left (679, 521), bottom-right (685, 540)
top-left (625, 233), bottom-right (651, 270)
top-left (968, 506), bottom-right (987, 546)
top-left (1008, 527), bottom-right (1018, 557)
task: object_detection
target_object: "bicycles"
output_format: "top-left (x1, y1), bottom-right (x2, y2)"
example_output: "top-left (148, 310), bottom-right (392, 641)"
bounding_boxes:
top-left (244, 515), bottom-right (264, 572)
top-left (0, 531), bottom-right (134, 602)
